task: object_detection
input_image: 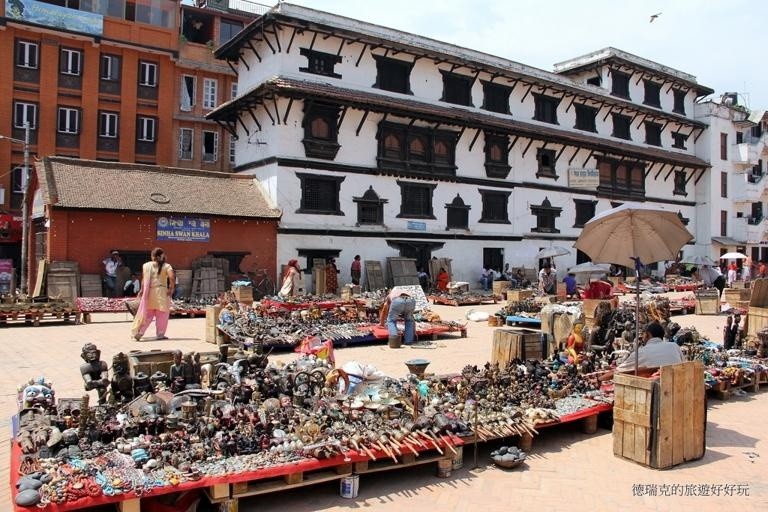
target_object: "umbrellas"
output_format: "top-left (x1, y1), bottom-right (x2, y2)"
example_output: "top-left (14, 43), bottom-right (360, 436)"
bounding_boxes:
top-left (679, 255), bottom-right (718, 266)
top-left (571, 202), bottom-right (695, 375)
top-left (536, 246), bottom-right (570, 268)
top-left (720, 252), bottom-right (748, 259)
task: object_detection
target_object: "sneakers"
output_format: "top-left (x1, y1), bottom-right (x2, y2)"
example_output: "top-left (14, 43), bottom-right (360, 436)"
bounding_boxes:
top-left (134, 335), bottom-right (169, 342)
top-left (389, 334), bottom-right (412, 349)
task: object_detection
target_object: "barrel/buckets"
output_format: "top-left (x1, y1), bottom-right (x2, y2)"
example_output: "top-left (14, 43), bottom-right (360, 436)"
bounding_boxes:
top-left (438, 457), bottom-right (453, 476)
top-left (340, 474), bottom-right (360, 499)
top-left (450, 445), bottom-right (464, 470)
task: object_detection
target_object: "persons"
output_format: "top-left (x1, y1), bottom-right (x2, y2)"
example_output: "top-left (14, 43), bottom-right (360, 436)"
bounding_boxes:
top-left (566, 321), bottom-right (589, 365)
top-left (726, 258), bottom-right (768, 284)
top-left (278, 257), bottom-right (302, 297)
top-left (325, 254), bottom-right (363, 292)
top-left (481, 263), bottom-right (582, 301)
top-left (691, 266), bottom-right (726, 295)
top-left (18, 378), bottom-right (55, 413)
top-left (378, 287), bottom-right (417, 348)
top-left (100, 247), bottom-right (175, 341)
top-left (167, 351), bottom-right (202, 386)
top-left (416, 265), bottom-right (450, 293)
top-left (81, 341), bottom-right (113, 387)
top-left (592, 319), bottom-right (687, 380)
top-left (249, 340), bottom-right (269, 369)
